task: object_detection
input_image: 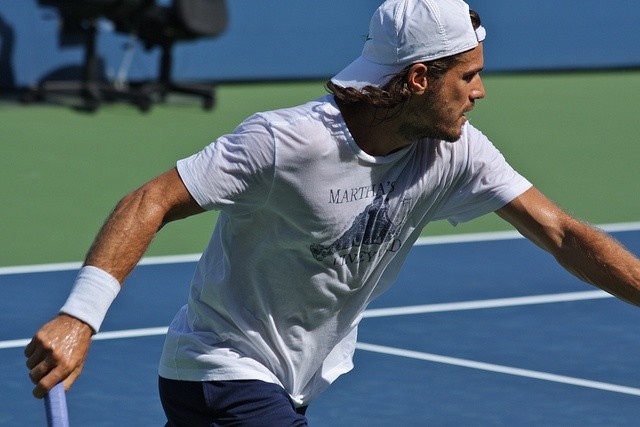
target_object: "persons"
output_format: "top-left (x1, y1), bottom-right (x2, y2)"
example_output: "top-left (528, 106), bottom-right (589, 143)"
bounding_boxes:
top-left (23, 1), bottom-right (640, 426)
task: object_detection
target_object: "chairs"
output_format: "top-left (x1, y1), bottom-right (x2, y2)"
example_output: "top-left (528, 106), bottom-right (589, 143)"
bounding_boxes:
top-left (125, 0), bottom-right (230, 111)
top-left (20, 0), bottom-right (124, 113)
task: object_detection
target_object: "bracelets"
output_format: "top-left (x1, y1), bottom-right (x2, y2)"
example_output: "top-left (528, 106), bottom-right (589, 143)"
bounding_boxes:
top-left (59, 263), bottom-right (123, 335)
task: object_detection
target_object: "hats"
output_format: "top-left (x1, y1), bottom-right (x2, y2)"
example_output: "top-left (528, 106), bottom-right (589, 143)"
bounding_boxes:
top-left (330, 0), bottom-right (488, 96)
